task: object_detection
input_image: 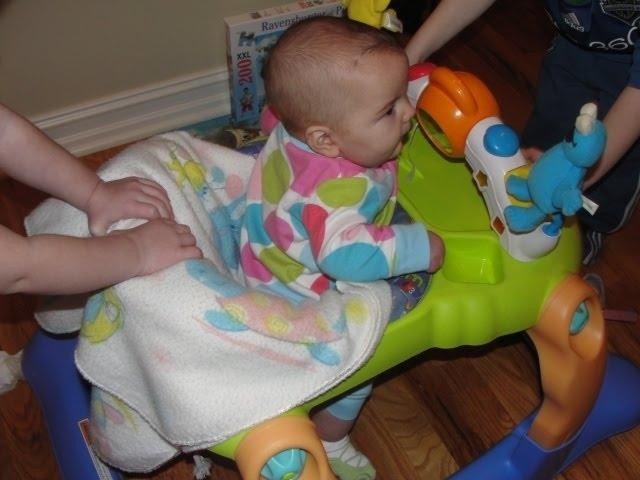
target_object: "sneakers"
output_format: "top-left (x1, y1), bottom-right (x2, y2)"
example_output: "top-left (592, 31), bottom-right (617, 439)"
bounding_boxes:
top-left (327, 439), bottom-right (376, 480)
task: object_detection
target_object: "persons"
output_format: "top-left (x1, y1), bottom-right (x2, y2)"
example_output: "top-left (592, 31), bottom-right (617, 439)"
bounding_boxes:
top-left (2, 100), bottom-right (206, 308)
top-left (406, 0), bottom-right (640, 204)
top-left (234, 15), bottom-right (417, 480)
